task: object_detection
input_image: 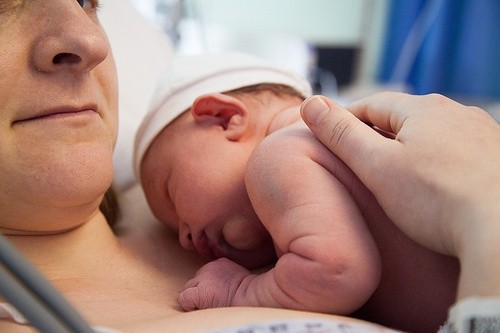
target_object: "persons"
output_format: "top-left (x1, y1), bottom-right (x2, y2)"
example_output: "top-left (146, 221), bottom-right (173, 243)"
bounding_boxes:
top-left (0, 1), bottom-right (500, 333)
top-left (133, 66), bottom-right (461, 332)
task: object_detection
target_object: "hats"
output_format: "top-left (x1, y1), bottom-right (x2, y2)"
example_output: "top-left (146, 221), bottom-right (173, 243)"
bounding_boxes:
top-left (130, 61), bottom-right (313, 183)
